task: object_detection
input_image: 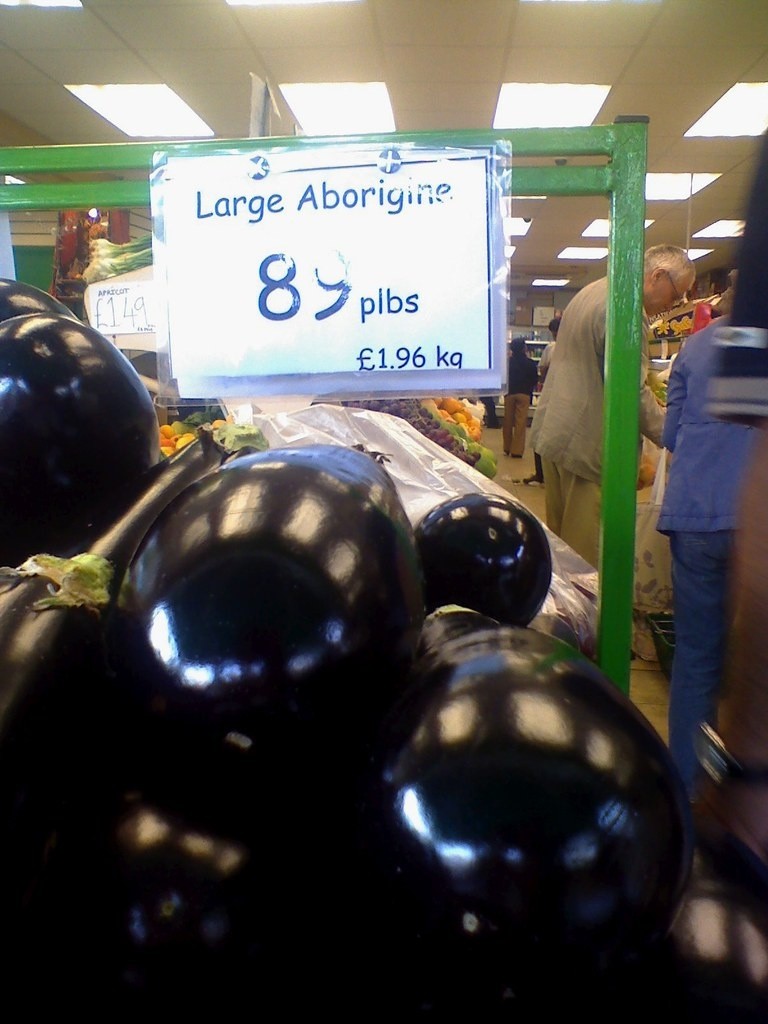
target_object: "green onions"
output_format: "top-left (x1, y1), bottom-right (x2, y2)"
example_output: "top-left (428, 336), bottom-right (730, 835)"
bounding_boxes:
top-left (81, 232), bottom-right (152, 282)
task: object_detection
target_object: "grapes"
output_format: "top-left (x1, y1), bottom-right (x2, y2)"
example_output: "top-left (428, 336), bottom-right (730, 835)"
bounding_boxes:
top-left (345, 398), bottom-right (482, 468)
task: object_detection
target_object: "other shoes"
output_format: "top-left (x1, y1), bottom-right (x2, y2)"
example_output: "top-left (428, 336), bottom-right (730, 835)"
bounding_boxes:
top-left (503, 451), bottom-right (523, 459)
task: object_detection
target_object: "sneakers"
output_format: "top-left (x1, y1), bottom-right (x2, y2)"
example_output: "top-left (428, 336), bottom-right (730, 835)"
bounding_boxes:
top-left (523, 475), bottom-right (545, 488)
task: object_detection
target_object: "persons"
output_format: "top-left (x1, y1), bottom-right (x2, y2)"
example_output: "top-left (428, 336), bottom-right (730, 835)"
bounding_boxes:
top-left (502, 338), bottom-right (538, 459)
top-left (527, 245), bottom-right (697, 564)
top-left (539, 317), bottom-right (560, 384)
top-left (709, 129), bottom-right (768, 858)
top-left (480, 397), bottom-right (500, 429)
top-left (661, 315), bottom-right (762, 795)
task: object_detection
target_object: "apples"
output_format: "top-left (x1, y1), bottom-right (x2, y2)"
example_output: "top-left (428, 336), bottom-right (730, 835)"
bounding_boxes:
top-left (159, 420), bottom-right (228, 457)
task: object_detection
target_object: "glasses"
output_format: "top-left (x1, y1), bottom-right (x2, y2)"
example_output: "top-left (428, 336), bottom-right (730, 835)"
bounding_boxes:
top-left (665, 271), bottom-right (683, 302)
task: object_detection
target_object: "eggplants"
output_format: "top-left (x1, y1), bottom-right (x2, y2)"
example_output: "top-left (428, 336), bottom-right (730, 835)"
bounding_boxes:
top-left (0, 444), bottom-right (768, 1024)
top-left (0, 278), bottom-right (160, 571)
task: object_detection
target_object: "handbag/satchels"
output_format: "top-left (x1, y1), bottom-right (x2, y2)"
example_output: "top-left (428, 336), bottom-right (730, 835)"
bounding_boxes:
top-left (634, 445), bottom-right (675, 615)
